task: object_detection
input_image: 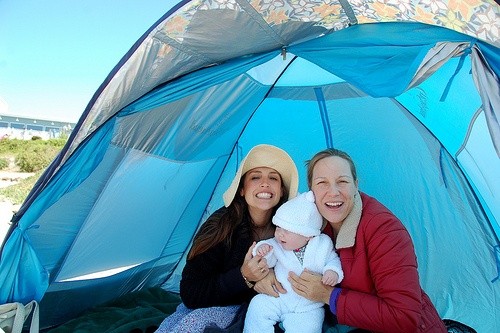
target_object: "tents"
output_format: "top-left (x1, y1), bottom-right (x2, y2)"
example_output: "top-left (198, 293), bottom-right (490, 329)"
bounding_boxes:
top-left (0, 0), bottom-right (500, 333)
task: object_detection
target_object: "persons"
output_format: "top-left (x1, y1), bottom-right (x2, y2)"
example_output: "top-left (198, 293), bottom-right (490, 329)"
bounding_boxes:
top-left (288, 148), bottom-right (448, 333)
top-left (153, 144), bottom-right (299, 333)
top-left (242, 191), bottom-right (344, 333)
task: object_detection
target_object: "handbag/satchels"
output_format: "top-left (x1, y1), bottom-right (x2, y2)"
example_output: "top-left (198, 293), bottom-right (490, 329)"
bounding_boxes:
top-left (0, 301), bottom-right (39, 333)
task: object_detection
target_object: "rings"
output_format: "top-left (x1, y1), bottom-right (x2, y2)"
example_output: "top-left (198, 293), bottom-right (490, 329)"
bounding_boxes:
top-left (259, 268), bottom-right (265, 273)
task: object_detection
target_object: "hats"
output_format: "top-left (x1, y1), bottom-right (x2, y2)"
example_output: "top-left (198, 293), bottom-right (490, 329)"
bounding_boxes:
top-left (222, 144), bottom-right (299, 208)
top-left (272, 190), bottom-right (322, 237)
